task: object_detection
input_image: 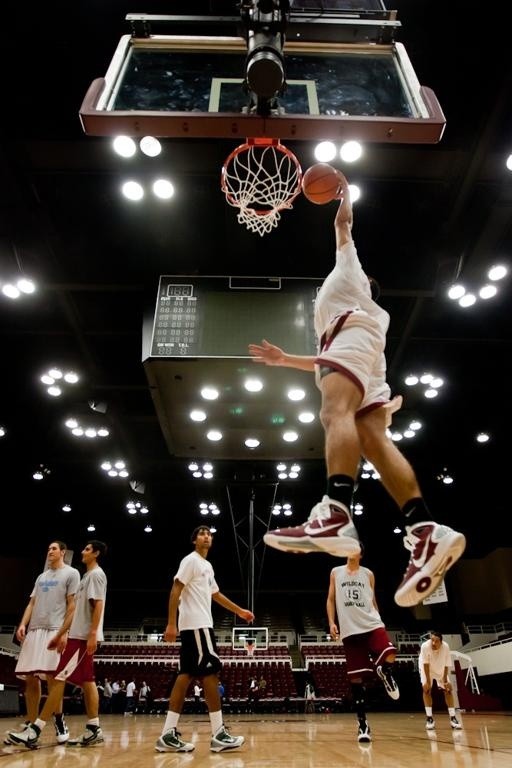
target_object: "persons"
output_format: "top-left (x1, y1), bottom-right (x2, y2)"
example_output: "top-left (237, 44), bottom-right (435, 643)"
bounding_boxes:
top-left (304, 681), bottom-right (316, 714)
top-left (72, 674), bottom-right (267, 717)
top-left (6, 540), bottom-right (108, 750)
top-left (0, 542), bottom-right (81, 750)
top-left (154, 525), bottom-right (256, 754)
top-left (246, 168), bottom-right (468, 609)
top-left (325, 539), bottom-right (403, 743)
top-left (417, 629), bottom-right (463, 731)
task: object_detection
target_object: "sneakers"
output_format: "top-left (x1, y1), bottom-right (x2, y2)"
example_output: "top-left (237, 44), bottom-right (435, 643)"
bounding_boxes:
top-left (53, 712), bottom-right (70, 746)
top-left (262, 494), bottom-right (362, 559)
top-left (3, 721), bottom-right (41, 750)
top-left (155, 726), bottom-right (195, 753)
top-left (449, 716), bottom-right (463, 730)
top-left (210, 724), bottom-right (245, 753)
top-left (67, 723), bottom-right (104, 747)
top-left (426, 715), bottom-right (436, 730)
top-left (376, 665), bottom-right (400, 701)
top-left (357, 719), bottom-right (372, 743)
top-left (394, 521), bottom-right (467, 608)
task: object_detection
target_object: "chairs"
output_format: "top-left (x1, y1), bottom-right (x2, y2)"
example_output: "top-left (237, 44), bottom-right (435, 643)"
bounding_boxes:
top-left (1, 632), bottom-right (512, 702)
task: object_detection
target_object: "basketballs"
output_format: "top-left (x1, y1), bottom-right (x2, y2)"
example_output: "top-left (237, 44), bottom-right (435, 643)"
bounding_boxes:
top-left (302, 163), bottom-right (339, 204)
